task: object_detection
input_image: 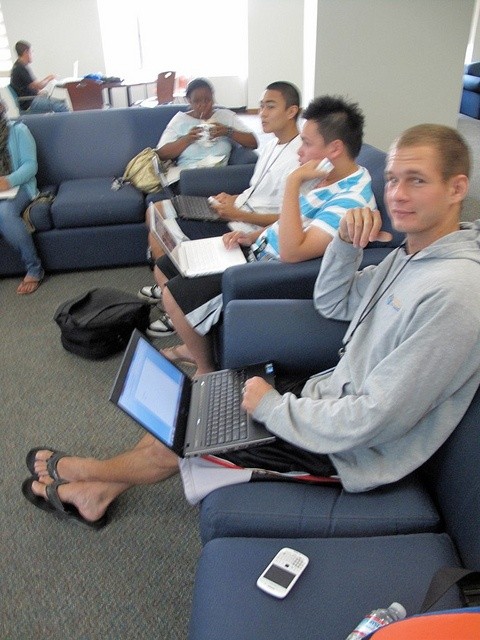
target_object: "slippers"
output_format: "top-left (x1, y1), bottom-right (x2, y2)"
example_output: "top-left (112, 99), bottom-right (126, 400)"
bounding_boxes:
top-left (27, 446), bottom-right (72, 480)
top-left (16, 269), bottom-right (45, 294)
top-left (21, 476), bottom-right (110, 529)
top-left (159, 344), bottom-right (198, 368)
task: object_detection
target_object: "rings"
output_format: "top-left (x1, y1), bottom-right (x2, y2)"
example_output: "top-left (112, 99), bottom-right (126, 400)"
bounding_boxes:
top-left (241, 386), bottom-right (246, 393)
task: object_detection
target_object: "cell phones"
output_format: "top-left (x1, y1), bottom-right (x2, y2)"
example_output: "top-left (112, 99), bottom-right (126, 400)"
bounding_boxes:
top-left (256, 545), bottom-right (310, 598)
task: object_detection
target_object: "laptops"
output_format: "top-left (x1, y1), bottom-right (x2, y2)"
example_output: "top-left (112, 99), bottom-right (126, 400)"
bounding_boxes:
top-left (151, 155), bottom-right (224, 222)
top-left (108, 327), bottom-right (279, 459)
top-left (57, 59), bottom-right (80, 83)
top-left (148, 200), bottom-right (248, 280)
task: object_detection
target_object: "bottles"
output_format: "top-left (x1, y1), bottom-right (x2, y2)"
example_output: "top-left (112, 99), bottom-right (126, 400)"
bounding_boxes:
top-left (343, 603), bottom-right (407, 640)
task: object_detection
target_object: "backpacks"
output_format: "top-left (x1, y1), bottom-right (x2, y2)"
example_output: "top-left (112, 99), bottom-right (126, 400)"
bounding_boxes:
top-left (53, 287), bottom-right (151, 360)
top-left (361, 568), bottom-right (480, 640)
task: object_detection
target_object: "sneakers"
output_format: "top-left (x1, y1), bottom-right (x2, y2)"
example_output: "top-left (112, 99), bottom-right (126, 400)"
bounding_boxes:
top-left (137, 283), bottom-right (162, 303)
top-left (146, 312), bottom-right (177, 338)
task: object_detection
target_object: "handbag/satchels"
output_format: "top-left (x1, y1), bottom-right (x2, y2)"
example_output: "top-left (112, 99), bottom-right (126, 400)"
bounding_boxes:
top-left (118, 147), bottom-right (172, 194)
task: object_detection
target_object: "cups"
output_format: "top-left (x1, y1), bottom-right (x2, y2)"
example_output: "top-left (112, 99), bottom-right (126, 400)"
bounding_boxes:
top-left (197, 124), bottom-right (216, 148)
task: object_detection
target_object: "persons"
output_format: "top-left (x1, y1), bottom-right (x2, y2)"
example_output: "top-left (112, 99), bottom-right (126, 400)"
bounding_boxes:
top-left (20, 124), bottom-right (480, 530)
top-left (0, 96), bottom-right (46, 295)
top-left (10, 40), bottom-right (70, 115)
top-left (138, 81), bottom-right (304, 337)
top-left (146, 78), bottom-right (257, 270)
top-left (158, 94), bottom-right (378, 377)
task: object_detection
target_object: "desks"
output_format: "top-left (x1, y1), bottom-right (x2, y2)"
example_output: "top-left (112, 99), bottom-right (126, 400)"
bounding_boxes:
top-left (56, 78), bottom-right (156, 107)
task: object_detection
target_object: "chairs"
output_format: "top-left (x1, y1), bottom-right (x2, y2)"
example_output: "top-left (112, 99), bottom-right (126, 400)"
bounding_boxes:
top-left (65, 79), bottom-right (104, 111)
top-left (6, 83), bottom-right (52, 114)
top-left (157, 71), bottom-right (176, 105)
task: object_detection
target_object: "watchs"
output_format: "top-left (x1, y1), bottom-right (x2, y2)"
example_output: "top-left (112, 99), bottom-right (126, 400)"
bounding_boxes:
top-left (226, 127), bottom-right (234, 138)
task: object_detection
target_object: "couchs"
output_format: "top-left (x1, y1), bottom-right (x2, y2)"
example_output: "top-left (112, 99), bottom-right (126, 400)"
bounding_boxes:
top-left (0, 104), bottom-right (259, 278)
top-left (460, 61), bottom-right (480, 119)
top-left (179, 143), bottom-right (405, 370)
top-left (190, 298), bottom-right (480, 639)
